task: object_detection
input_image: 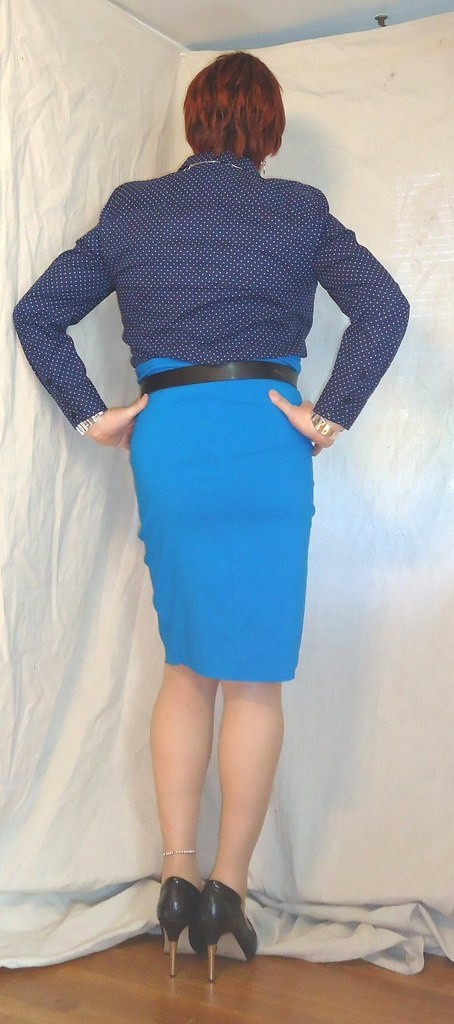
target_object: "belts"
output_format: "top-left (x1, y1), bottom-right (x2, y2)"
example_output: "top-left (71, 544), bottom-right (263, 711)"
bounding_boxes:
top-left (138, 359), bottom-right (298, 400)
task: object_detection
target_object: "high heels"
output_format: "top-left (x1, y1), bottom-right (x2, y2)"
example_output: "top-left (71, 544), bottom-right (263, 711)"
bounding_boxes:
top-left (196, 879), bottom-right (258, 982)
top-left (156, 876), bottom-right (204, 977)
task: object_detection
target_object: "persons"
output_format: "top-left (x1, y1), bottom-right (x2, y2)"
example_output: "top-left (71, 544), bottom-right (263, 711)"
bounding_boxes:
top-left (12, 49), bottom-right (411, 983)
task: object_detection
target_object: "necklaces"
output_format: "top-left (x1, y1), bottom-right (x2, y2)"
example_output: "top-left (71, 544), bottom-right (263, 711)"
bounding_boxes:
top-left (186, 160), bottom-right (242, 171)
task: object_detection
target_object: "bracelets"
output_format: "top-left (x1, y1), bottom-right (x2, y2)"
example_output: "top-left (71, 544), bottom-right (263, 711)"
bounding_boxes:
top-left (311, 413), bottom-right (341, 440)
top-left (76, 410), bottom-right (103, 436)
